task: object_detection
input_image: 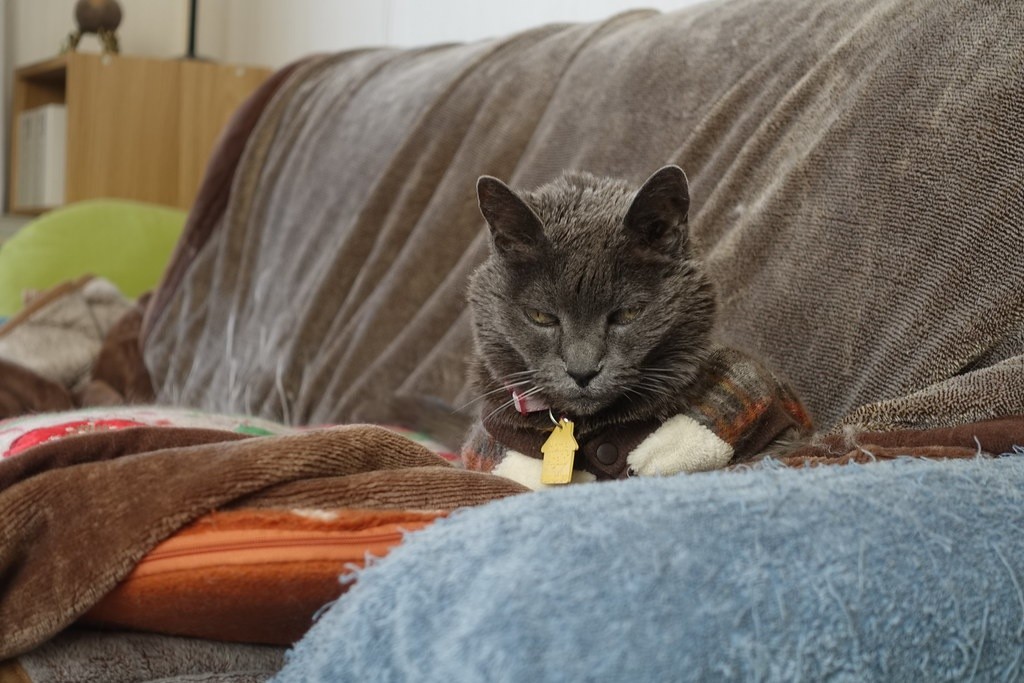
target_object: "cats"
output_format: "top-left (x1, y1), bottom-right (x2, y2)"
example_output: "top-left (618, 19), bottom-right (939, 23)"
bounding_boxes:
top-left (461, 166), bottom-right (813, 490)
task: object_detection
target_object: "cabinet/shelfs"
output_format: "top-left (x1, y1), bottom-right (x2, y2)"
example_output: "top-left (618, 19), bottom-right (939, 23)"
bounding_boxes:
top-left (7, 49), bottom-right (272, 213)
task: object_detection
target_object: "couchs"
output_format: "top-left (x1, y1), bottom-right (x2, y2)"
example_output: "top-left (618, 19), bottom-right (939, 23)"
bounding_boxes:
top-left (2, 1), bottom-right (1024, 683)
